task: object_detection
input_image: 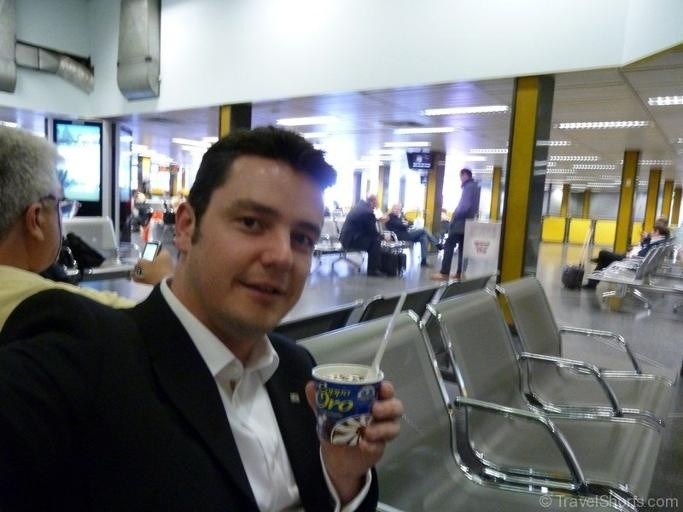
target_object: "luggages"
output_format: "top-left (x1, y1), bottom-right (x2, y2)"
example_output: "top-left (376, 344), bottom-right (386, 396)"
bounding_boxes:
top-left (561, 230), bottom-right (593, 290)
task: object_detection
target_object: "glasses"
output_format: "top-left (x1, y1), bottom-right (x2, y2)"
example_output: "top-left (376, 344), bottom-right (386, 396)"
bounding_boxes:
top-left (35, 196), bottom-right (82, 219)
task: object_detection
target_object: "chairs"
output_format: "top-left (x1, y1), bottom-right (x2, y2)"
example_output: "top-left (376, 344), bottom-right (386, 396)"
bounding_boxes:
top-left (60, 217), bottom-right (140, 285)
top-left (313, 217), bottom-right (403, 270)
top-left (595, 235), bottom-right (676, 320)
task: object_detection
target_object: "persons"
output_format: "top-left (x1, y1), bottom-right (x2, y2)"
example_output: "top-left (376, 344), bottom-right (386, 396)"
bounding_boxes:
top-left (591, 217), bottom-right (670, 265)
top-left (0, 124), bottom-right (403, 511)
top-left (383, 200), bottom-right (447, 269)
top-left (579, 220), bottom-right (669, 292)
top-left (337, 193), bottom-right (394, 277)
top-left (0, 121), bottom-right (173, 351)
top-left (429, 168), bottom-right (481, 281)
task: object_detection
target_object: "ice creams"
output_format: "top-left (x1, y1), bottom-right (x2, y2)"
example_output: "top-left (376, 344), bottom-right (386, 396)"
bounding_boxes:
top-left (318, 373), bottom-right (365, 381)
top-left (331, 416), bottom-right (373, 447)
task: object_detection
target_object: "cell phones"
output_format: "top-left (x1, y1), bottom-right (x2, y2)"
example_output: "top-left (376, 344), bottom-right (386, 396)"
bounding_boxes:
top-left (134, 239), bottom-right (163, 276)
